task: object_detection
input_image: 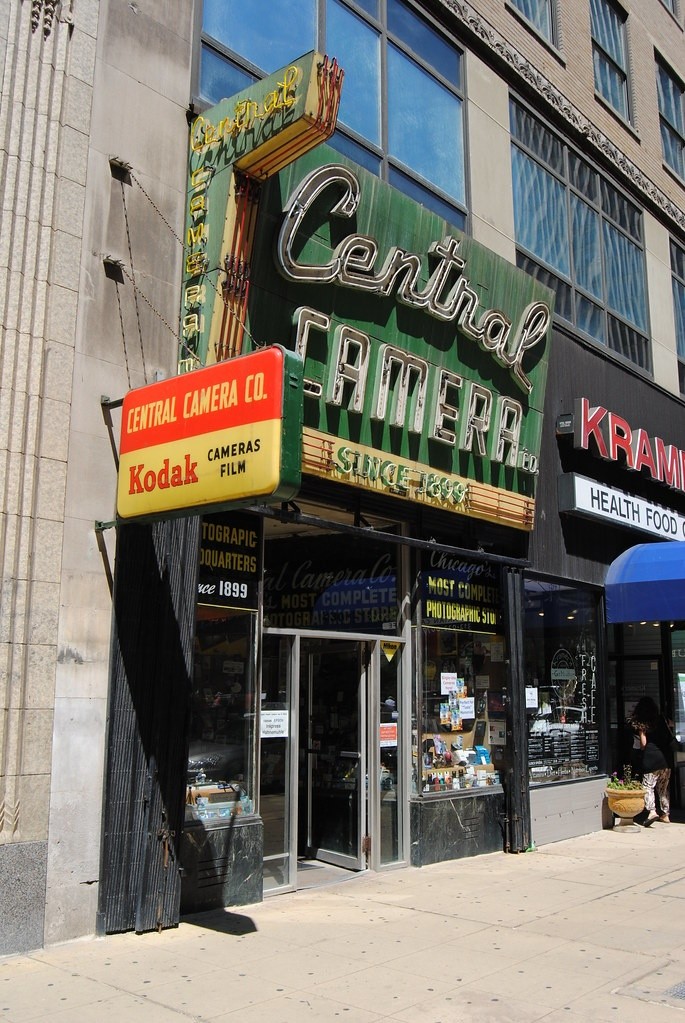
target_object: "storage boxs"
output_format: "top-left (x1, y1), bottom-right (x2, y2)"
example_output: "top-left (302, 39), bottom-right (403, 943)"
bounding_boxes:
top-left (454, 747), bottom-right (490, 765)
top-left (476, 770), bottom-right (487, 788)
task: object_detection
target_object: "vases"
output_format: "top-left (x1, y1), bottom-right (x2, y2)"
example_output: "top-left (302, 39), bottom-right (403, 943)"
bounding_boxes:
top-left (605, 788), bottom-right (647, 817)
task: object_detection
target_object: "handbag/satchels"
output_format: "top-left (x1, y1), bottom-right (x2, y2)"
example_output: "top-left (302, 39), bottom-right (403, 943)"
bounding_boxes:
top-left (670, 739), bottom-right (679, 753)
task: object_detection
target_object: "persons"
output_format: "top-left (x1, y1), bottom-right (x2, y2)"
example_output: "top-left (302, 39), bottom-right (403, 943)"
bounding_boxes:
top-left (628, 697), bottom-right (676, 828)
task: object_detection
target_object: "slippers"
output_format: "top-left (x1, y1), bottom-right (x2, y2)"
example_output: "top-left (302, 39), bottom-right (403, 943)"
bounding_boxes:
top-left (659, 819), bottom-right (670, 823)
top-left (643, 815), bottom-right (659, 827)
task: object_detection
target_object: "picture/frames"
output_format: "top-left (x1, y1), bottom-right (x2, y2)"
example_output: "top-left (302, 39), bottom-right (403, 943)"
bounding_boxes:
top-left (436, 631), bottom-right (458, 655)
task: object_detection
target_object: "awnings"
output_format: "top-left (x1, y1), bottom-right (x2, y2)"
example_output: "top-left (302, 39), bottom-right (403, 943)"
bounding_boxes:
top-left (606, 540), bottom-right (684, 623)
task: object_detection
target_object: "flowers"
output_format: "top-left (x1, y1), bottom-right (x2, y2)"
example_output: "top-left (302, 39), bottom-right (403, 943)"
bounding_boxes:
top-left (607, 764), bottom-right (644, 790)
